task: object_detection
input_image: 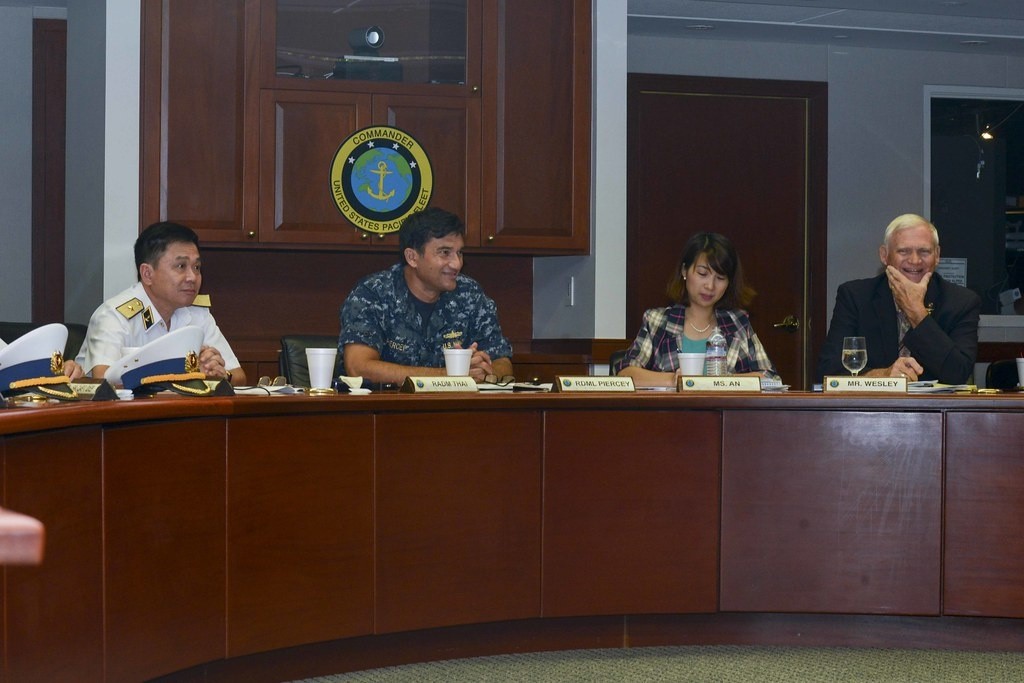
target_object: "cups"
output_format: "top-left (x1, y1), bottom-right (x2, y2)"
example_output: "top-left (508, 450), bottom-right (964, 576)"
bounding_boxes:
top-left (677, 353), bottom-right (705, 374)
top-left (443, 349), bottom-right (472, 376)
top-left (305, 348), bottom-right (337, 389)
top-left (121, 347), bottom-right (140, 358)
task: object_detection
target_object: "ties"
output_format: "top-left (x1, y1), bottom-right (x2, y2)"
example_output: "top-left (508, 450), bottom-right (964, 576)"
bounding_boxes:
top-left (899, 313), bottom-right (911, 367)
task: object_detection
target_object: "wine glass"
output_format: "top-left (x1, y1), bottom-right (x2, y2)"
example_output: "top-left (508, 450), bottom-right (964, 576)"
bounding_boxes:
top-left (842, 337), bottom-right (867, 377)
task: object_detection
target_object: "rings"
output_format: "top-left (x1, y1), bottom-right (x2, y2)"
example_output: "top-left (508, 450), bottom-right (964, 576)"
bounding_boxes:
top-left (901, 374), bottom-right (905, 377)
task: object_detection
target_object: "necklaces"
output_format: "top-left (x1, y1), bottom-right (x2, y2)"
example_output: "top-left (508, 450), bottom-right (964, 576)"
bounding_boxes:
top-left (690, 322), bottom-right (710, 333)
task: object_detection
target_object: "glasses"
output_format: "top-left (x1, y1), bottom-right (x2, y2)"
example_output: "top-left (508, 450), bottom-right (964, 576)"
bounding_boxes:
top-left (484, 375), bottom-right (515, 387)
top-left (257, 376), bottom-right (286, 387)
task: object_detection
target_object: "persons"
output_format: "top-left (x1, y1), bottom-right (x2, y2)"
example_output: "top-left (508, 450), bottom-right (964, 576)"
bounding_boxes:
top-left (820, 214), bottom-right (982, 386)
top-left (75, 222), bottom-right (247, 388)
top-left (616, 232), bottom-right (780, 387)
top-left (336, 208), bottom-right (514, 386)
top-left (0, 337), bottom-right (84, 382)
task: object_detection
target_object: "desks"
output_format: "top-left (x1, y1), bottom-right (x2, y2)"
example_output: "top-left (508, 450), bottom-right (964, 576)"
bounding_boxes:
top-left (0, 388), bottom-right (1024, 683)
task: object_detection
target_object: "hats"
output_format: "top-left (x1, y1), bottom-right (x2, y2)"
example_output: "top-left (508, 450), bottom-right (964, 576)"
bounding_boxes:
top-left (0, 323), bottom-right (80, 401)
top-left (103, 325), bottom-right (216, 396)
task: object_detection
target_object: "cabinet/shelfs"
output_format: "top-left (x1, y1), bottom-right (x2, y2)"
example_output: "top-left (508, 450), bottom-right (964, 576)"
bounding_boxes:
top-left (138, 0), bottom-right (592, 257)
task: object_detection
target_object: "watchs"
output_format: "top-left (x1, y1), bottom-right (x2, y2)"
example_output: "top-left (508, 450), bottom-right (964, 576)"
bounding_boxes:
top-left (227, 371), bottom-right (232, 382)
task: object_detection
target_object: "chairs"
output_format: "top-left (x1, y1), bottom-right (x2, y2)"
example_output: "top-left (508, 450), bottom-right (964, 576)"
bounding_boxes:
top-left (277, 334), bottom-right (339, 388)
top-left (986, 360), bottom-right (1020, 391)
top-left (0, 322), bottom-right (88, 362)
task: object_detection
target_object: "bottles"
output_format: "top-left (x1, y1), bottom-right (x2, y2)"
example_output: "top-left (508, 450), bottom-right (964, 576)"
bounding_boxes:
top-left (706, 327), bottom-right (727, 374)
